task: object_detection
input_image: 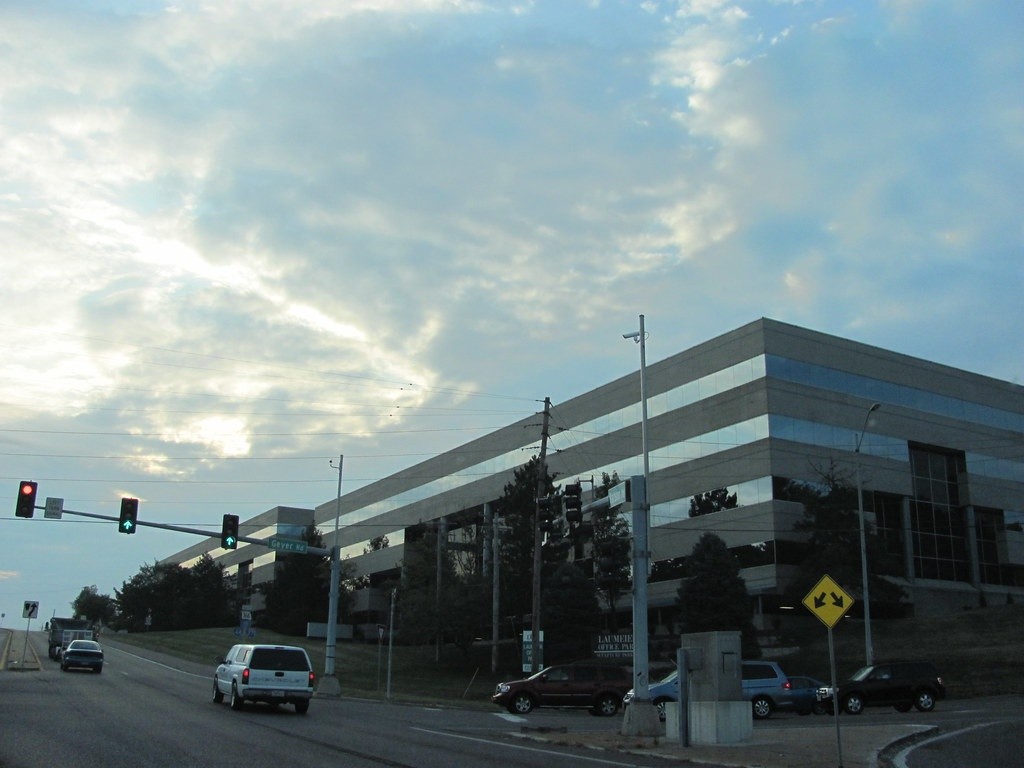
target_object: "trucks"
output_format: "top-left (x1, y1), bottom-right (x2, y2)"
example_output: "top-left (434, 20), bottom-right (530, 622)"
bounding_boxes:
top-left (43, 616), bottom-right (100, 663)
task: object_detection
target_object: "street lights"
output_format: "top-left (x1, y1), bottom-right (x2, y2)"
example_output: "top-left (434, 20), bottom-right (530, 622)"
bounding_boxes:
top-left (854, 400), bottom-right (881, 667)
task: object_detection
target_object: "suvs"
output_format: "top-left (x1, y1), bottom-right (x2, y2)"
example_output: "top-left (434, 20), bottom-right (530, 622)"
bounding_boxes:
top-left (491, 662), bottom-right (633, 718)
top-left (621, 659), bottom-right (795, 720)
top-left (815, 658), bottom-right (950, 716)
top-left (211, 642), bottom-right (317, 712)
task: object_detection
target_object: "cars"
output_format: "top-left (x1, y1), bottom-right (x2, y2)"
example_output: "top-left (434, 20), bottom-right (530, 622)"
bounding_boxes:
top-left (789, 675), bottom-right (832, 716)
top-left (59, 639), bottom-right (104, 674)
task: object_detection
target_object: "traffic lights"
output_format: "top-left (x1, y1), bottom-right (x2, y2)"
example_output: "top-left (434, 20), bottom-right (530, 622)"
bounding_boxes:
top-left (118, 497), bottom-right (139, 534)
top-left (559, 476), bottom-right (582, 529)
top-left (220, 514), bottom-right (239, 550)
top-left (535, 493), bottom-right (557, 533)
top-left (15, 481), bottom-right (38, 518)
